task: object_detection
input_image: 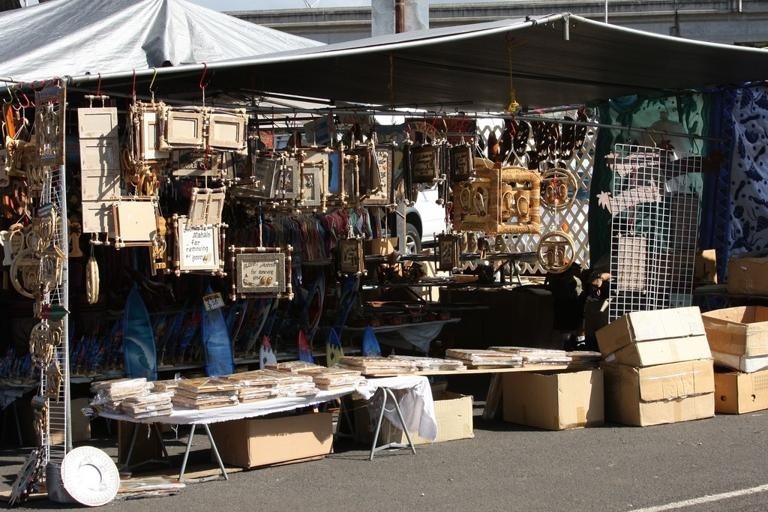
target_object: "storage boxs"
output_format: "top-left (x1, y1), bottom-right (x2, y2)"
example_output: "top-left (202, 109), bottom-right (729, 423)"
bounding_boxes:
top-left (502, 255), bottom-right (768, 431)
top-left (210, 412), bottom-right (335, 470)
top-left (381, 389), bottom-right (474, 446)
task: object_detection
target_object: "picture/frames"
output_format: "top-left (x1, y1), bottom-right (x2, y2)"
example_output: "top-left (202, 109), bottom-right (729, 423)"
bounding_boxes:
top-left (77, 92), bottom-right (471, 295)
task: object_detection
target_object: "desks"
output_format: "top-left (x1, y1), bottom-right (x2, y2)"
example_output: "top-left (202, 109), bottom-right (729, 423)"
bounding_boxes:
top-left (94, 375), bottom-right (429, 485)
